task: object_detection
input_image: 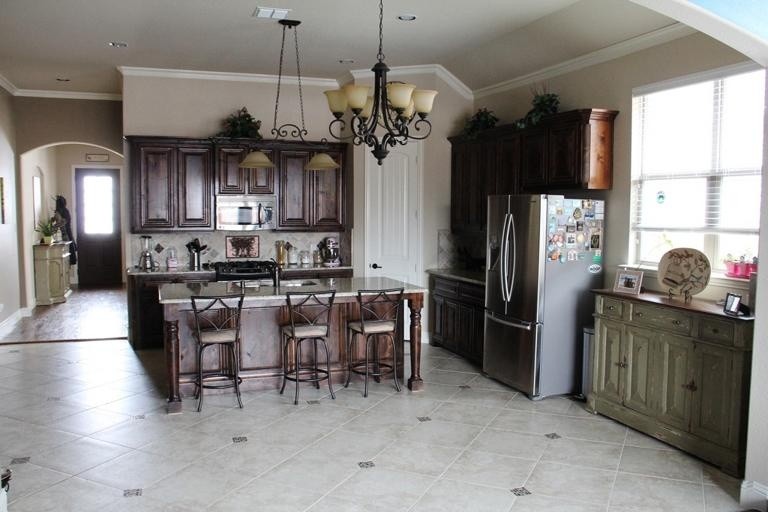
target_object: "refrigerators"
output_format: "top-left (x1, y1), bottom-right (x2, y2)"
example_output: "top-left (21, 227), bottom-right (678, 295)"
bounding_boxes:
top-left (483, 194), bottom-right (607, 401)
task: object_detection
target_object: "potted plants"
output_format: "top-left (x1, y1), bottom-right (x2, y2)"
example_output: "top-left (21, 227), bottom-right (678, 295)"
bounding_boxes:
top-left (36, 217), bottom-right (63, 245)
top-left (517, 92), bottom-right (559, 130)
top-left (458, 109), bottom-right (500, 144)
top-left (221, 105), bottom-right (263, 139)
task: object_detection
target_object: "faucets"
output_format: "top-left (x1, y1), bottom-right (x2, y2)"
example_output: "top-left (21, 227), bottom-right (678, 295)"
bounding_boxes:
top-left (273, 266), bottom-right (280, 286)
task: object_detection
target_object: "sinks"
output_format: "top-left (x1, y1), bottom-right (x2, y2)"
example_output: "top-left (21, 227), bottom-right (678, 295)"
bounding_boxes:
top-left (233, 279), bottom-right (317, 286)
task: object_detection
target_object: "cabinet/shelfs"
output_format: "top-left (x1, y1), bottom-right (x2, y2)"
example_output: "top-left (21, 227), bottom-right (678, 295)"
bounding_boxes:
top-left (431, 277), bottom-right (485, 366)
top-left (123, 133), bottom-right (351, 234)
top-left (447, 108), bottom-right (620, 236)
top-left (584, 289), bottom-right (756, 478)
top-left (36, 241), bottom-right (74, 306)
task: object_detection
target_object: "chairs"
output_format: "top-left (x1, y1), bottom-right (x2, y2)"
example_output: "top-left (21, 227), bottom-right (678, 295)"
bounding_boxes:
top-left (279, 286), bottom-right (405, 405)
top-left (189, 294), bottom-right (248, 412)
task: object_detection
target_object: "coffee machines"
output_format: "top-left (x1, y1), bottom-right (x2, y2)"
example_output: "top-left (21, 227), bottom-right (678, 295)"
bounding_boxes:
top-left (137, 235), bottom-right (160, 271)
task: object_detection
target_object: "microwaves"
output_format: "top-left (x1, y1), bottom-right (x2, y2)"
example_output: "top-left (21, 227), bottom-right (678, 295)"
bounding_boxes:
top-left (215, 194), bottom-right (278, 231)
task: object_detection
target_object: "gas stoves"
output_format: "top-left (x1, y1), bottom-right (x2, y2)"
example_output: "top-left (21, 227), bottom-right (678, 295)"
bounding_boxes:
top-left (204, 260), bottom-right (273, 270)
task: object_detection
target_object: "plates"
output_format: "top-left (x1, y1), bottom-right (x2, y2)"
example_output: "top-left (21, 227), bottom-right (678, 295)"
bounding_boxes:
top-left (658, 247), bottom-right (712, 296)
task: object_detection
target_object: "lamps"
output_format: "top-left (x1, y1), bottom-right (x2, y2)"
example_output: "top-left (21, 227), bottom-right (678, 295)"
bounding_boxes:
top-left (236, 1), bottom-right (439, 172)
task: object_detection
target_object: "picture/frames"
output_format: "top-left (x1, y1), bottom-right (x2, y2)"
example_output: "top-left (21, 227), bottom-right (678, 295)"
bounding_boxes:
top-left (611, 268), bottom-right (644, 295)
top-left (724, 292), bottom-right (743, 316)
top-left (225, 235), bottom-right (261, 259)
